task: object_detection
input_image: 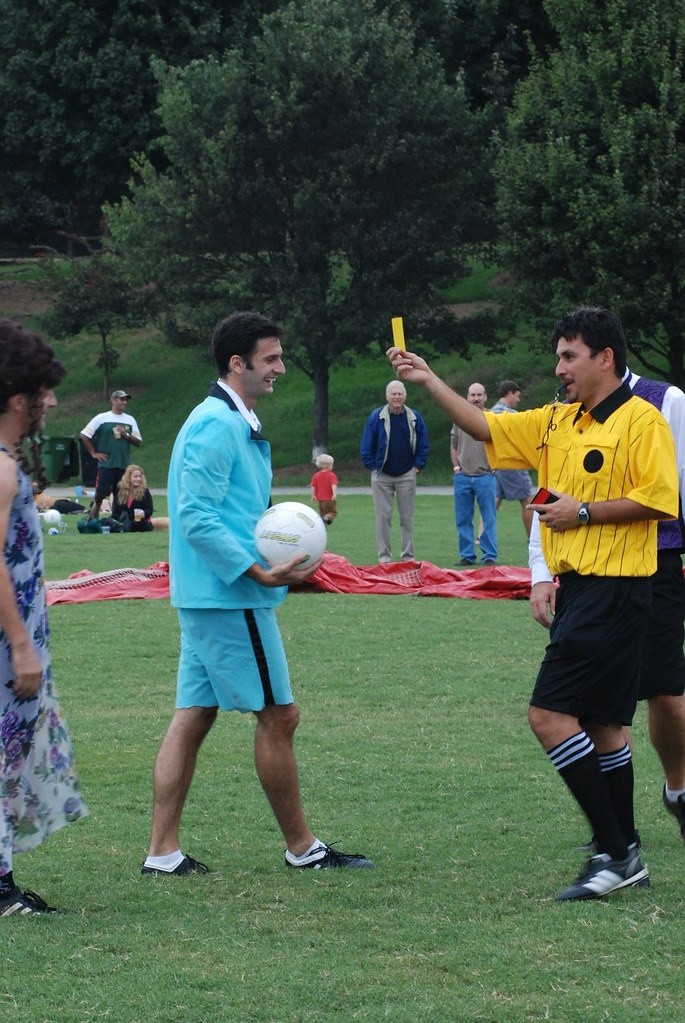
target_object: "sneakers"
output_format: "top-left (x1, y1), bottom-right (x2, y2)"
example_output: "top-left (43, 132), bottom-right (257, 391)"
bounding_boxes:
top-left (141, 854), bottom-right (209, 877)
top-left (284, 840), bottom-right (375, 871)
top-left (662, 783), bottom-right (685, 834)
top-left (556, 840), bottom-right (648, 902)
top-left (0, 886), bottom-right (58, 915)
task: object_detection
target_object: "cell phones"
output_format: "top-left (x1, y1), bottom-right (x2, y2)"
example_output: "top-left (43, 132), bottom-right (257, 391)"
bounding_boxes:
top-left (531, 487), bottom-right (560, 515)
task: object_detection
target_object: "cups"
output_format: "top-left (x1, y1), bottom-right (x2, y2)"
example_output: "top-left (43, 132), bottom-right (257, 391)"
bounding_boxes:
top-left (58, 522), bottom-right (67, 533)
top-left (101, 526), bottom-right (111, 534)
top-left (112, 427), bottom-right (121, 439)
top-left (134, 509), bottom-right (141, 522)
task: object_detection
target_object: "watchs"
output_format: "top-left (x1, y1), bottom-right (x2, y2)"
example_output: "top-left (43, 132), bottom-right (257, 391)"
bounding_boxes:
top-left (578, 502), bottom-right (589, 527)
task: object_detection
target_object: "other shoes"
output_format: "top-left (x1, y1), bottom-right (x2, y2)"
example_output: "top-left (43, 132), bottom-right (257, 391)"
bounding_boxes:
top-left (485, 559), bottom-right (492, 566)
top-left (454, 559), bottom-right (472, 566)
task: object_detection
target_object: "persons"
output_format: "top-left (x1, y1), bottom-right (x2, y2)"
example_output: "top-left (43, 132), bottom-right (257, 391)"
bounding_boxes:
top-left (386, 306), bottom-right (680, 904)
top-left (79, 390), bottom-right (169, 534)
top-left (526, 363), bottom-right (685, 845)
top-left (311, 454), bottom-right (339, 525)
top-left (489, 381), bottom-right (532, 538)
top-left (449, 382), bottom-right (499, 567)
top-left (361, 380), bottom-right (430, 564)
top-left (138, 311), bottom-right (375, 875)
top-left (0, 321), bottom-right (91, 917)
top-left (31, 481), bottom-right (89, 513)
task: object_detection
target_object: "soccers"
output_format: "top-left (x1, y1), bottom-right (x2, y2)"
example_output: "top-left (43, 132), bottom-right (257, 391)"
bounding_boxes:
top-left (44, 509), bottom-right (62, 524)
top-left (255, 501), bottom-right (328, 572)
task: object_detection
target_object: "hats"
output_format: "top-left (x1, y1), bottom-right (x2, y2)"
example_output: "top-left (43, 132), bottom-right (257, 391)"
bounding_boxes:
top-left (112, 390), bottom-right (131, 400)
